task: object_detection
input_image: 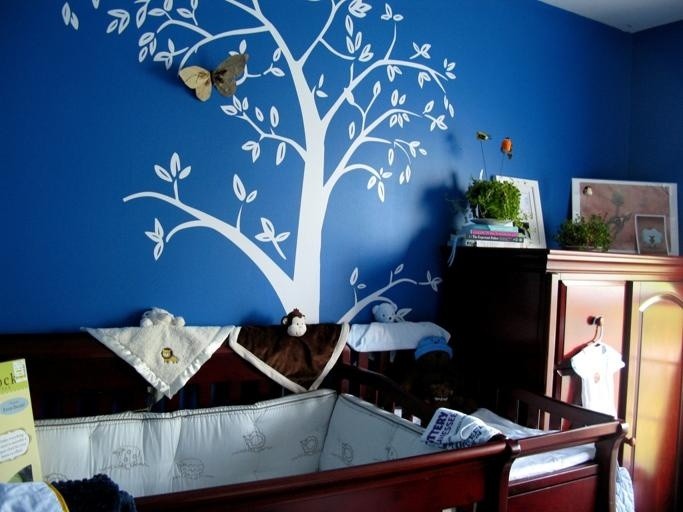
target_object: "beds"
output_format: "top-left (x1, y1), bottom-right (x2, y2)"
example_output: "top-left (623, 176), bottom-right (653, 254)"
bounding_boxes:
top-left (0, 320), bottom-right (637, 511)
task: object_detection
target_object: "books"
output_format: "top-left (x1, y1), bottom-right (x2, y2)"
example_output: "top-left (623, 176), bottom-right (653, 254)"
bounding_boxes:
top-left (448, 225), bottom-right (527, 248)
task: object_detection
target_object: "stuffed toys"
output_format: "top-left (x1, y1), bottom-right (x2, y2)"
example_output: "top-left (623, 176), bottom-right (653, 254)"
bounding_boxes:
top-left (281, 308), bottom-right (306, 337)
top-left (372, 303), bottom-right (397, 322)
top-left (140, 307), bottom-right (185, 327)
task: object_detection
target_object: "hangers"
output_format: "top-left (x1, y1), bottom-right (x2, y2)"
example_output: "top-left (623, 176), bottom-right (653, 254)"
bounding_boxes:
top-left (588, 318), bottom-right (606, 348)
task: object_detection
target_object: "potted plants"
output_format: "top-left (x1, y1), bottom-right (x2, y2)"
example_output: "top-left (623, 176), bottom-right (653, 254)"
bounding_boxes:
top-left (441, 168), bottom-right (534, 232)
top-left (553, 211), bottom-right (623, 253)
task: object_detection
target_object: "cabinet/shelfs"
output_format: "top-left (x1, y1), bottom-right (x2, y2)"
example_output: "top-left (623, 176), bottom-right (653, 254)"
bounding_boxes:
top-left (444, 242), bottom-right (682, 511)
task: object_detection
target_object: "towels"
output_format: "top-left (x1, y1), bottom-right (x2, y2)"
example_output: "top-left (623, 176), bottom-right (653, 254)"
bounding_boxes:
top-left (80, 324), bottom-right (237, 399)
top-left (228, 323), bottom-right (350, 394)
top-left (419, 404), bottom-right (505, 452)
top-left (346, 320), bottom-right (451, 353)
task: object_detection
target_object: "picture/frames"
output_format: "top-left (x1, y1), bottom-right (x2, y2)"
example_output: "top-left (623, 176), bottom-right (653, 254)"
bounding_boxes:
top-left (570, 177), bottom-right (679, 257)
